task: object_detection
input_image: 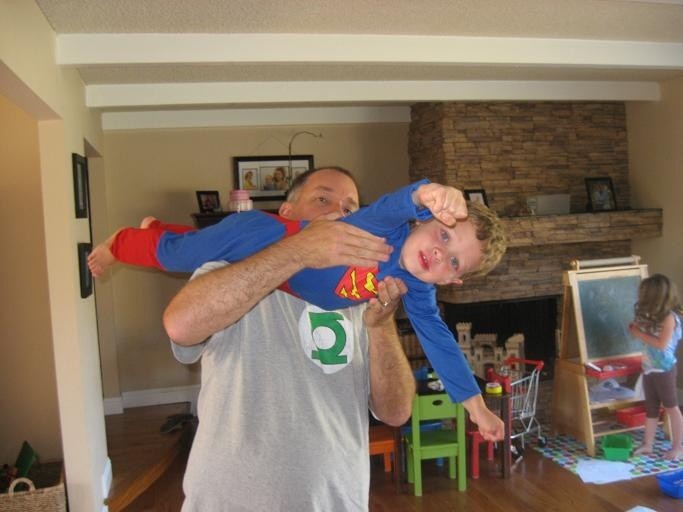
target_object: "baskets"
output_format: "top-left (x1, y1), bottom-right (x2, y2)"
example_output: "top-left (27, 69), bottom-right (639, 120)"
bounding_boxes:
top-left (0, 453), bottom-right (68, 511)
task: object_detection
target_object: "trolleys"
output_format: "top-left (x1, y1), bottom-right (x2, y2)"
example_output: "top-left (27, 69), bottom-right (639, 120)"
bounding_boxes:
top-left (493, 354), bottom-right (547, 463)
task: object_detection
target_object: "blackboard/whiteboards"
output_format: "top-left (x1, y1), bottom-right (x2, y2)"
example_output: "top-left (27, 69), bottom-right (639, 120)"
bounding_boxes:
top-left (571, 268), bottom-right (649, 366)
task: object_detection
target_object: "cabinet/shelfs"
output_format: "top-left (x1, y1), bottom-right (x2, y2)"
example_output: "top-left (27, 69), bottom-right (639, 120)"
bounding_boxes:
top-left (107, 401), bottom-right (199, 512)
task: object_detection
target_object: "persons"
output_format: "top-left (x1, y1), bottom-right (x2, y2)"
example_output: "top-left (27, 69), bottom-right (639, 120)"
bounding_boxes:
top-left (244, 167), bottom-right (289, 190)
top-left (162, 167), bottom-right (417, 512)
top-left (204, 195), bottom-right (217, 210)
top-left (594, 183), bottom-right (610, 210)
top-left (87, 179), bottom-right (509, 443)
top-left (630, 275), bottom-right (682, 459)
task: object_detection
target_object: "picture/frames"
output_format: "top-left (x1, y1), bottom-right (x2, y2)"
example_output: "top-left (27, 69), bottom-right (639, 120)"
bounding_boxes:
top-left (232, 152), bottom-right (314, 203)
top-left (72, 152), bottom-right (88, 218)
top-left (196, 190), bottom-right (220, 212)
top-left (77, 242), bottom-right (92, 298)
top-left (464, 188), bottom-right (489, 209)
top-left (584, 176), bottom-right (617, 212)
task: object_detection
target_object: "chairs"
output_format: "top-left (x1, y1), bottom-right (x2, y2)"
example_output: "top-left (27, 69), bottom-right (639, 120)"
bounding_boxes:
top-left (466, 425), bottom-right (494, 480)
top-left (405, 393), bottom-right (467, 497)
top-left (369, 423), bottom-right (399, 479)
top-left (398, 364), bottom-right (445, 469)
